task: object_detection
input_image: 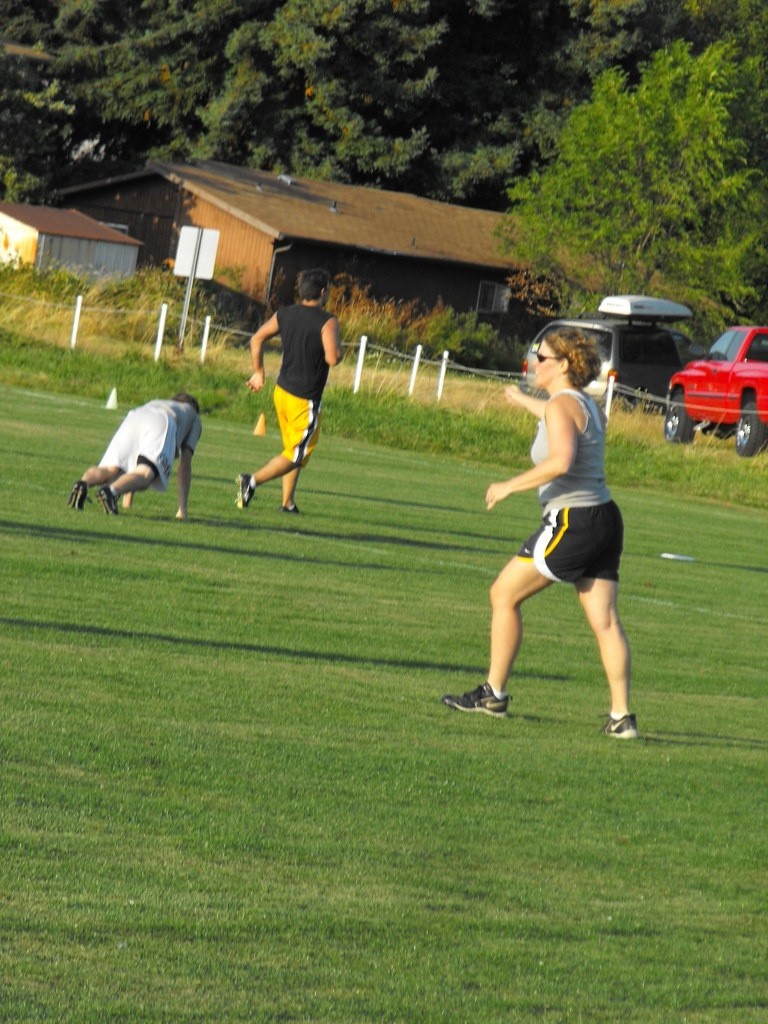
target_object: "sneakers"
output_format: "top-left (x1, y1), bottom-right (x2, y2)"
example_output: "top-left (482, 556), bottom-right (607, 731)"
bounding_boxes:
top-left (599, 712), bottom-right (639, 739)
top-left (281, 504), bottom-right (301, 515)
top-left (67, 480), bottom-right (88, 511)
top-left (94, 482), bottom-right (120, 516)
top-left (441, 682), bottom-right (514, 718)
top-left (235, 472), bottom-right (256, 510)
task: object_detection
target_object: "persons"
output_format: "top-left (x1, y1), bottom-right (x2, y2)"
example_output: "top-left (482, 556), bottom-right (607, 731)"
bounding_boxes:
top-left (234, 268), bottom-right (344, 514)
top-left (67, 393), bottom-right (202, 519)
top-left (442, 326), bottom-right (640, 738)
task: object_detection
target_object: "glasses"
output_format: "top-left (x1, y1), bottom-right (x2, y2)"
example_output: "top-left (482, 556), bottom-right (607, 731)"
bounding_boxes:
top-left (535, 352), bottom-right (564, 365)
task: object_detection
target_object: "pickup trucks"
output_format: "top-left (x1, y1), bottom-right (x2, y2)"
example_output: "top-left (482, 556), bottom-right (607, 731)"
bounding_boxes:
top-left (662, 324), bottom-right (768, 458)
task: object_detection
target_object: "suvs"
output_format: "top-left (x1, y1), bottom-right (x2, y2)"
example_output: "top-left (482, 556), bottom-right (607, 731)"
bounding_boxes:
top-left (517, 295), bottom-right (703, 416)
